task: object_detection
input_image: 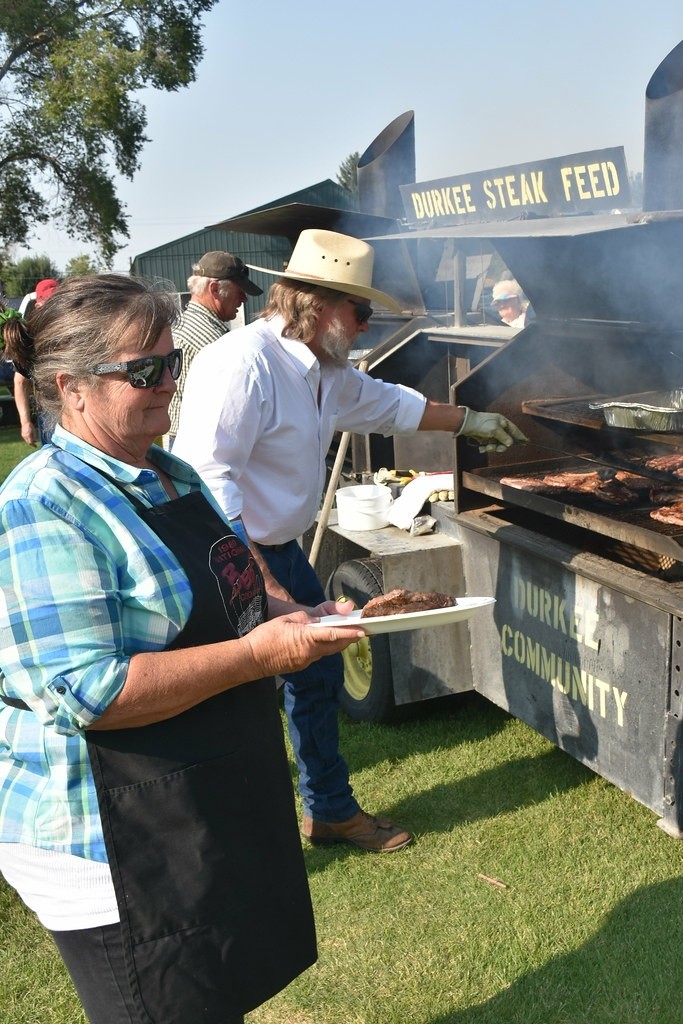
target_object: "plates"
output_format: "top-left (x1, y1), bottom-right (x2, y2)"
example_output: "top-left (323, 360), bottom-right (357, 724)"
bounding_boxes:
top-left (305, 596), bottom-right (497, 636)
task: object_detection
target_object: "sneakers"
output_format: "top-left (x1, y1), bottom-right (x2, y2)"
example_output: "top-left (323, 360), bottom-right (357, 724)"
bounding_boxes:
top-left (301, 809), bottom-right (412, 851)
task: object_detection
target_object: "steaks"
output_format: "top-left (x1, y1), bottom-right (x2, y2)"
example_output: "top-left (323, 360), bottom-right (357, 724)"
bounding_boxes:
top-left (360, 588), bottom-right (457, 619)
top-left (500, 452), bottom-right (683, 528)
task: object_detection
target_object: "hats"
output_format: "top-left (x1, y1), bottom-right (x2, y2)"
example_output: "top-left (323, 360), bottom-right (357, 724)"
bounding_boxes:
top-left (35, 280), bottom-right (61, 305)
top-left (490, 279), bottom-right (518, 306)
top-left (246, 228), bottom-right (402, 314)
top-left (192, 251), bottom-right (265, 296)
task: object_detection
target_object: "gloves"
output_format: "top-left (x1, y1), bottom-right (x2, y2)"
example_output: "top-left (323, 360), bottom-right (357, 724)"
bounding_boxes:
top-left (451, 405), bottom-right (529, 453)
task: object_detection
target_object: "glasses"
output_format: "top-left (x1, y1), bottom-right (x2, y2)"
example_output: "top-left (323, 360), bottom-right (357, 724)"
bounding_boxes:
top-left (346, 299), bottom-right (373, 324)
top-left (86, 348), bottom-right (184, 389)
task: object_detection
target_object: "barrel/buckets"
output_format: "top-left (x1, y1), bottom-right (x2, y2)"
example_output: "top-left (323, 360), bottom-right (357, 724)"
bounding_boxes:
top-left (334, 484), bottom-right (395, 532)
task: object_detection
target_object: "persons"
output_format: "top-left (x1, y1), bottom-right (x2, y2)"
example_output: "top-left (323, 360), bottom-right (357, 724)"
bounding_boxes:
top-left (166, 249), bottom-right (265, 453)
top-left (0, 272), bottom-right (366, 1024)
top-left (490, 281), bottom-right (530, 328)
top-left (168, 231), bottom-right (531, 852)
top-left (14, 278), bottom-right (62, 448)
